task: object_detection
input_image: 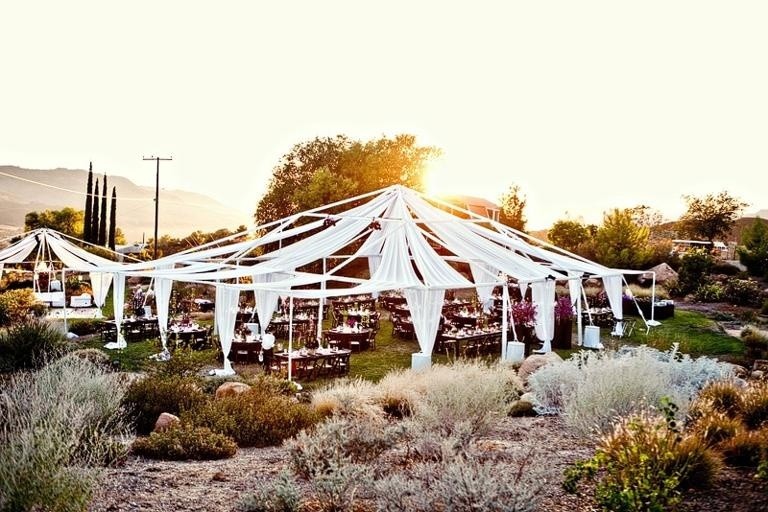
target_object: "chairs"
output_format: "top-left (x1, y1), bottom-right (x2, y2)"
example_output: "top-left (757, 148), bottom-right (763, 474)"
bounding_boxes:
top-left (383, 291), bottom-right (620, 361)
top-left (98, 294), bottom-right (381, 382)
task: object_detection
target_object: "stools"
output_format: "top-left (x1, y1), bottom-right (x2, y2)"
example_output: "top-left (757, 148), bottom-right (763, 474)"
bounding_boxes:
top-left (622, 317), bottom-right (637, 337)
